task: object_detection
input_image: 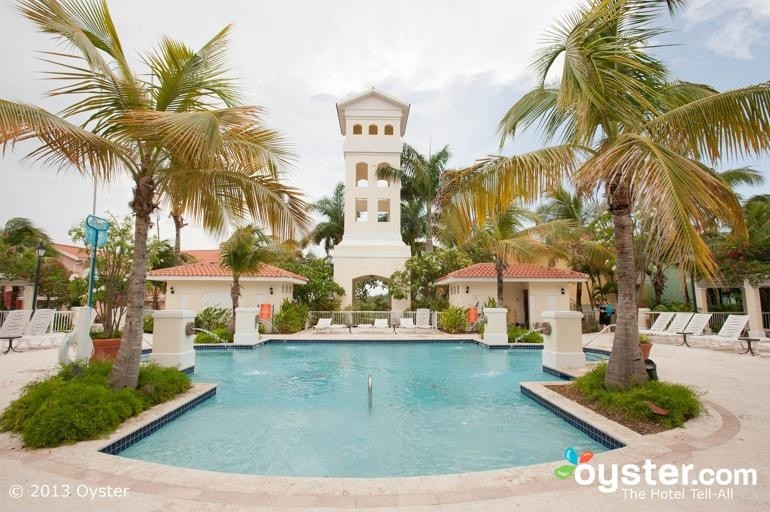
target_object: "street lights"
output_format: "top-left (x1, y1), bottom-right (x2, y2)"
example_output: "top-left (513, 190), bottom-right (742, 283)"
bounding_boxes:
top-left (31, 241), bottom-right (46, 311)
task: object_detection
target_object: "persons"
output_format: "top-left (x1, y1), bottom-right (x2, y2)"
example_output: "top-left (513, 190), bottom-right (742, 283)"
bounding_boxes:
top-left (598, 298), bottom-right (612, 325)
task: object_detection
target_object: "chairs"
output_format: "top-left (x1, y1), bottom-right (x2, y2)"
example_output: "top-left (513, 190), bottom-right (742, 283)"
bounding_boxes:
top-left (0, 308), bottom-right (55, 354)
top-left (312, 318), bottom-right (435, 334)
top-left (634, 312), bottom-right (770, 350)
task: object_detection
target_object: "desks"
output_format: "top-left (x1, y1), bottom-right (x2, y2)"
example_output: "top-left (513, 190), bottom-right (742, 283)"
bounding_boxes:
top-left (737, 337), bottom-right (760, 356)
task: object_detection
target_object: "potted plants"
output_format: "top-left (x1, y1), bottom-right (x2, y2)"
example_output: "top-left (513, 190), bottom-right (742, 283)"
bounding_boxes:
top-left (67, 210), bottom-right (171, 364)
top-left (638, 333), bottom-right (653, 359)
